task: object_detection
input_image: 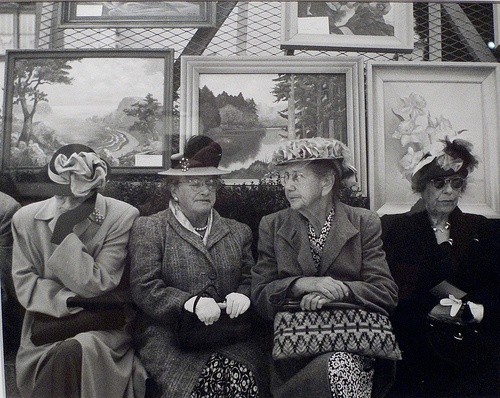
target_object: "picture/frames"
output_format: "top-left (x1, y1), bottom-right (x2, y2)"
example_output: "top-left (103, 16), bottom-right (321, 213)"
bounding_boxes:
top-left (0, 0), bottom-right (500, 221)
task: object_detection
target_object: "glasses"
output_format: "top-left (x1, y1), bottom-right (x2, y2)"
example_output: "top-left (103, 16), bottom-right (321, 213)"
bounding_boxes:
top-left (430, 177), bottom-right (465, 189)
top-left (176, 178), bottom-right (222, 192)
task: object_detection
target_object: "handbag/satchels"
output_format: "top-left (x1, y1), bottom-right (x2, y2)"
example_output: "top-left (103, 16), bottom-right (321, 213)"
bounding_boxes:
top-left (176, 284), bottom-right (255, 352)
top-left (271, 299), bottom-right (402, 362)
top-left (29, 304), bottom-right (127, 347)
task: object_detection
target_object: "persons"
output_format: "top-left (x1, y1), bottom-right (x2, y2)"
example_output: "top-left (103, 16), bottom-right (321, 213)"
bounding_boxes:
top-left (11, 143), bottom-right (155, 398)
top-left (381, 143), bottom-right (500, 398)
top-left (255, 138), bottom-right (399, 398)
top-left (0, 190), bottom-right (23, 398)
top-left (127, 154), bottom-right (267, 398)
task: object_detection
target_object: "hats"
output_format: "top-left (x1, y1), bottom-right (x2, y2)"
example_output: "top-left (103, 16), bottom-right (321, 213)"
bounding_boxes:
top-left (34, 143), bottom-right (112, 198)
top-left (157, 134), bottom-right (231, 176)
top-left (407, 138), bottom-right (478, 193)
top-left (272, 137), bottom-right (348, 167)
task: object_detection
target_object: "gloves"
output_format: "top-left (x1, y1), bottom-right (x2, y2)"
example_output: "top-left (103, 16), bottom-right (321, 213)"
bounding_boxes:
top-left (50, 191), bottom-right (98, 246)
top-left (68, 291), bottom-right (126, 312)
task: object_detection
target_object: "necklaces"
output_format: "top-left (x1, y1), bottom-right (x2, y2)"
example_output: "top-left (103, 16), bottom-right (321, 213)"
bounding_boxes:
top-left (433, 222), bottom-right (450, 233)
top-left (193, 224), bottom-right (208, 231)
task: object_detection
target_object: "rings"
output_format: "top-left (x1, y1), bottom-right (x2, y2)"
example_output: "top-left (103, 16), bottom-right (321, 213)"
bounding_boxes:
top-left (315, 294), bottom-right (321, 299)
